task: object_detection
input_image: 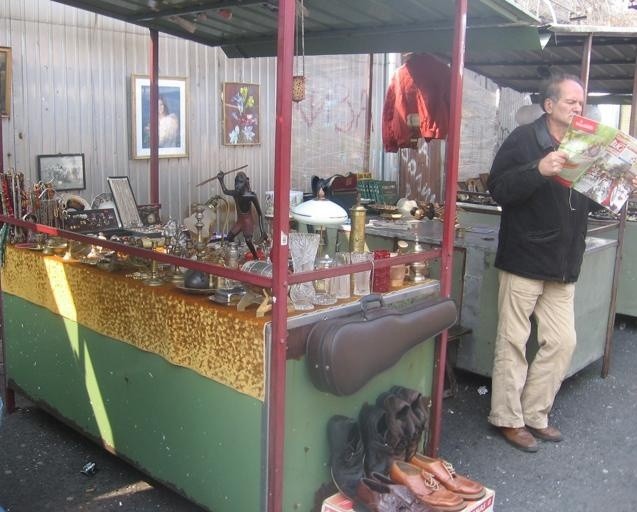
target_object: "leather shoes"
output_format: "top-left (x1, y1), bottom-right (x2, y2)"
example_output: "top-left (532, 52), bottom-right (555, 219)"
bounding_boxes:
top-left (329, 385), bottom-right (486, 511)
top-left (525, 424), bottom-right (564, 442)
top-left (498, 427), bottom-right (538, 452)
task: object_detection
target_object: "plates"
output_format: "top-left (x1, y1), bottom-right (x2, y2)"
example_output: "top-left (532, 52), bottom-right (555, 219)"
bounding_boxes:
top-left (370, 204), bottom-right (400, 219)
top-left (90, 193), bottom-right (125, 228)
top-left (175, 282), bottom-right (218, 295)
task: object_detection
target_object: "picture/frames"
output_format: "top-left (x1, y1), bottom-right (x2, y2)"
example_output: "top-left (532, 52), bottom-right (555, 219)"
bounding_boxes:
top-left (221, 82), bottom-right (260, 147)
top-left (0, 45), bottom-right (13, 120)
top-left (37, 153), bottom-right (85, 193)
top-left (129, 74), bottom-right (190, 160)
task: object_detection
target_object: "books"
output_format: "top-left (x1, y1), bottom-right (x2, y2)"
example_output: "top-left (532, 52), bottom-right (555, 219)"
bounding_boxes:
top-left (552, 112), bottom-right (637, 215)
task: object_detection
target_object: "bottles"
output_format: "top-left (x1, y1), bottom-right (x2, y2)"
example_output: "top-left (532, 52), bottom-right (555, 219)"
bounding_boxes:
top-left (225, 240), bottom-right (240, 266)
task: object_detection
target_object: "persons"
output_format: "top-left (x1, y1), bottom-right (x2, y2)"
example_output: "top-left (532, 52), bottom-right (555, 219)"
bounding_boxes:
top-left (155, 96), bottom-right (180, 147)
top-left (213, 171), bottom-right (267, 260)
top-left (486, 72), bottom-right (637, 451)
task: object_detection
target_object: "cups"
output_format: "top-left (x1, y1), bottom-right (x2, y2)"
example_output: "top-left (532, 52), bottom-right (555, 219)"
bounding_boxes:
top-left (288, 232), bottom-right (411, 312)
